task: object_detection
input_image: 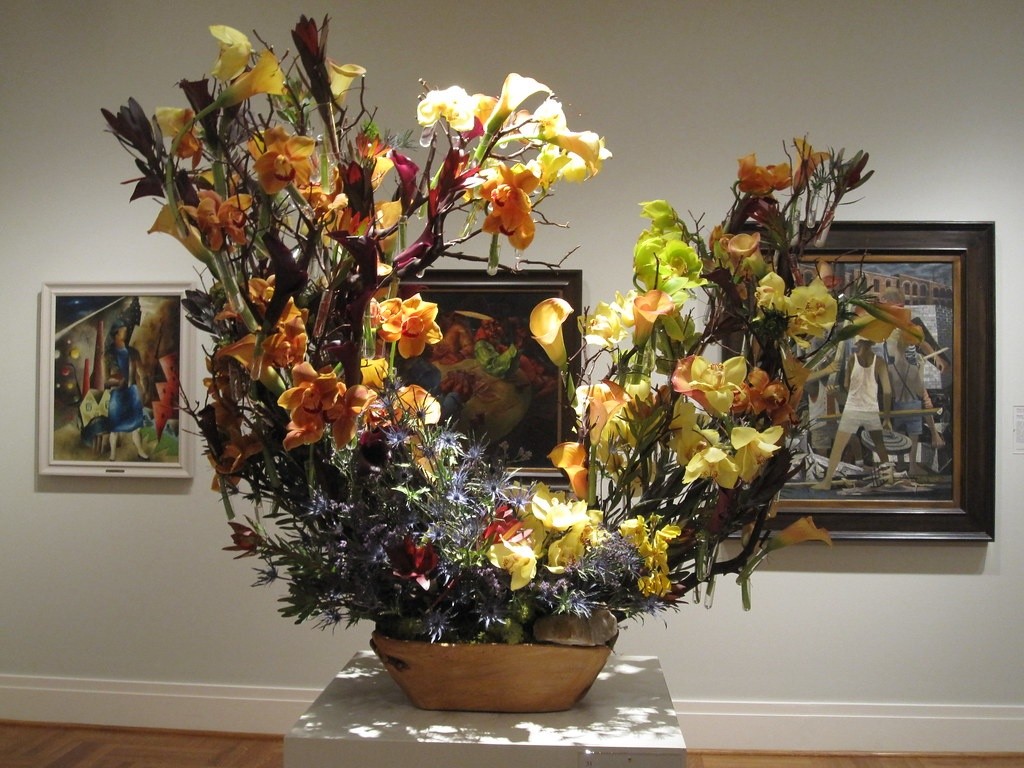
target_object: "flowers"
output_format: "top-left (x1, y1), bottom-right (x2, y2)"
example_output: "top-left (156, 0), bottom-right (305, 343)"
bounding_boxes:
top-left (97, 11), bottom-right (875, 645)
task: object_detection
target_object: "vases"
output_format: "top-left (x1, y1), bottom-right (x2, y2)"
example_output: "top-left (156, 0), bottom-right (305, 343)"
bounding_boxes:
top-left (368, 630), bottom-right (619, 713)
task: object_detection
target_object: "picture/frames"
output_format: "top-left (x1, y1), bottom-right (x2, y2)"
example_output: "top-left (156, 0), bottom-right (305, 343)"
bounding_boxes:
top-left (721, 224), bottom-right (996, 542)
top-left (37, 282), bottom-right (196, 479)
top-left (369, 269), bottom-right (584, 494)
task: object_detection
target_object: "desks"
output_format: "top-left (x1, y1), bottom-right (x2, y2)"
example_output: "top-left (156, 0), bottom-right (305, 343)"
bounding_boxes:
top-left (281, 647), bottom-right (686, 767)
